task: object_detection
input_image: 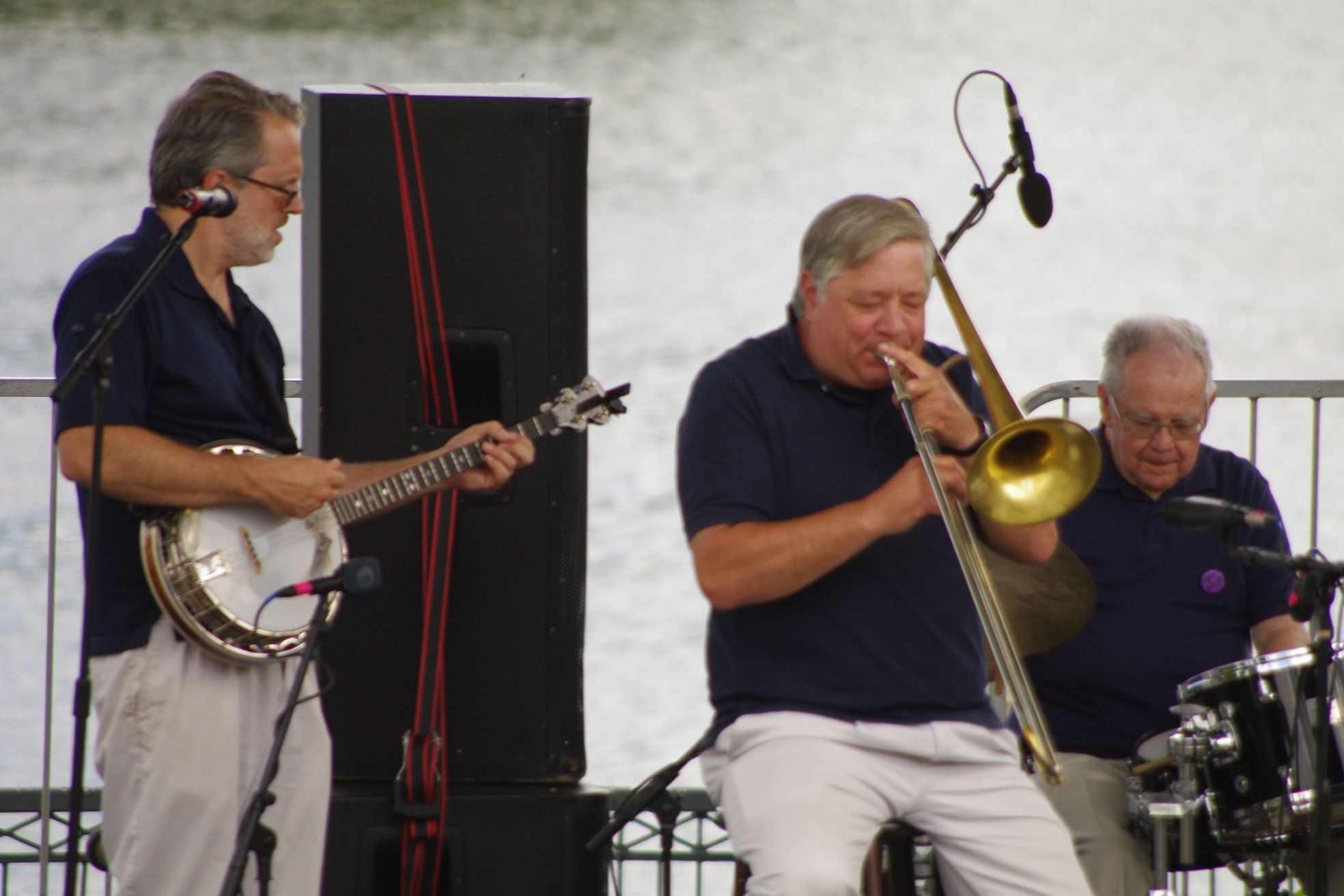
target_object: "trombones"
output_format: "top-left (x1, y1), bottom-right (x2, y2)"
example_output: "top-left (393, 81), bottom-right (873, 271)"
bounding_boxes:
top-left (877, 195), bottom-right (1102, 787)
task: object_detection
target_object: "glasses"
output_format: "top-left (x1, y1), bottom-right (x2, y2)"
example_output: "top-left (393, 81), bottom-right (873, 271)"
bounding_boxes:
top-left (1106, 390), bottom-right (1208, 440)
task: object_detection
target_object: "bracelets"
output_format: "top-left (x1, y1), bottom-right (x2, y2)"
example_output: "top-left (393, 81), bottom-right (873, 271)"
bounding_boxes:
top-left (937, 414), bottom-right (989, 456)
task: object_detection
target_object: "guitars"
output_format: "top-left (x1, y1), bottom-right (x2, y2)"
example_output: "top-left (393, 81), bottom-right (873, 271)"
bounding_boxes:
top-left (139, 377), bottom-right (633, 665)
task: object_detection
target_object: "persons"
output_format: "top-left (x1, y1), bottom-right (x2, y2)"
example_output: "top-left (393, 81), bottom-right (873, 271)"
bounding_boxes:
top-left (54, 71), bottom-right (536, 896)
top-left (675, 196), bottom-right (1098, 895)
top-left (1022, 314), bottom-right (1308, 896)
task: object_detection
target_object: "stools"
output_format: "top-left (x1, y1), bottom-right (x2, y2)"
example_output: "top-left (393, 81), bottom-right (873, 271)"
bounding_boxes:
top-left (714, 812), bottom-right (948, 896)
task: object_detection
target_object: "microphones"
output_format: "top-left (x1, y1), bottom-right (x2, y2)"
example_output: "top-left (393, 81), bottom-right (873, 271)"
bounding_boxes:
top-left (1002, 81), bottom-right (1053, 227)
top-left (1161, 496), bottom-right (1274, 531)
top-left (1288, 550), bottom-right (1319, 624)
top-left (173, 185), bottom-right (238, 218)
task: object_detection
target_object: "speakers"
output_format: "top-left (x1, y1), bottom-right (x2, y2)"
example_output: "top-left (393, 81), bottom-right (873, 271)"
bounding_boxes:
top-left (316, 781), bottom-right (611, 896)
top-left (296, 86), bottom-right (592, 784)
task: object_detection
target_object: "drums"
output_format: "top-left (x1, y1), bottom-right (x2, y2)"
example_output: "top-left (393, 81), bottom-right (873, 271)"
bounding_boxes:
top-left (1176, 639), bottom-right (1344, 842)
